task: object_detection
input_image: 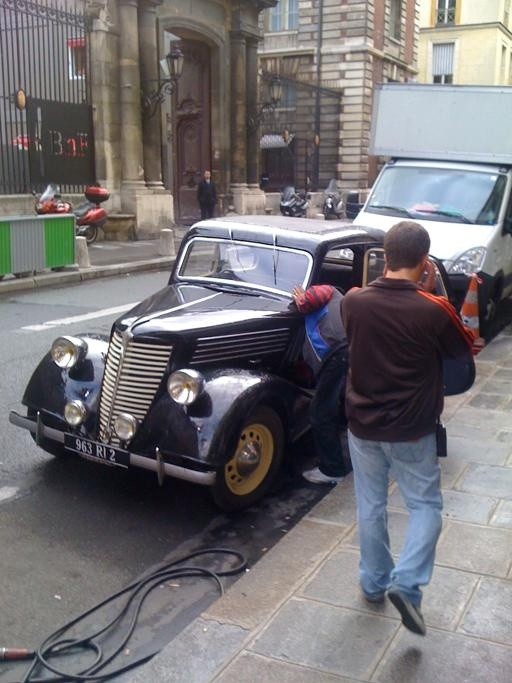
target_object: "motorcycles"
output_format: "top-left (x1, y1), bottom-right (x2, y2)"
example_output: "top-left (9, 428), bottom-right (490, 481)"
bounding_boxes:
top-left (279, 185), bottom-right (311, 216)
top-left (28, 180), bottom-right (110, 244)
top-left (323, 178), bottom-right (348, 220)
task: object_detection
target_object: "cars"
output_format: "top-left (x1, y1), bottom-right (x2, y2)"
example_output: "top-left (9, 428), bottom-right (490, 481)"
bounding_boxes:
top-left (2, 211), bottom-right (455, 514)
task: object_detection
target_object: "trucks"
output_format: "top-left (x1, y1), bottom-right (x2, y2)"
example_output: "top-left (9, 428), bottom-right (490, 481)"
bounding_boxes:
top-left (350, 81), bottom-right (512, 330)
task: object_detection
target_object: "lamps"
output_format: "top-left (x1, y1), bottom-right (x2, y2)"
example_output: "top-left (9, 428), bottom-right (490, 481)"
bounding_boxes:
top-left (247, 72), bottom-right (283, 137)
top-left (139, 42), bottom-right (186, 121)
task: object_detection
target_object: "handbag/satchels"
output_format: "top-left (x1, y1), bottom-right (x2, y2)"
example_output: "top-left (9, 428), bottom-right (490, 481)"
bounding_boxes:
top-left (435, 422), bottom-right (448, 457)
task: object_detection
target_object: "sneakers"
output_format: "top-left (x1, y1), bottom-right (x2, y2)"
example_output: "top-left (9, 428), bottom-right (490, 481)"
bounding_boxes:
top-left (386, 590), bottom-right (426, 637)
top-left (302, 466), bottom-right (343, 486)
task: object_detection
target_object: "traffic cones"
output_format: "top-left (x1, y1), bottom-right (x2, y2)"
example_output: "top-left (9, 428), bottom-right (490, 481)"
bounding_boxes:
top-left (457, 274), bottom-right (489, 346)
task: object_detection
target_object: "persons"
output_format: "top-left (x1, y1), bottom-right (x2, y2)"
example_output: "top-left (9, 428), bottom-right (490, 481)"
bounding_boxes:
top-left (338, 219), bottom-right (478, 637)
top-left (288, 279), bottom-right (351, 486)
top-left (196, 169), bottom-right (218, 220)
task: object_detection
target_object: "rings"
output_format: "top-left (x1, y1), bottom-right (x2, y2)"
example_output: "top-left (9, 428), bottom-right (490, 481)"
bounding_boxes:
top-left (296, 293), bottom-right (298, 295)
